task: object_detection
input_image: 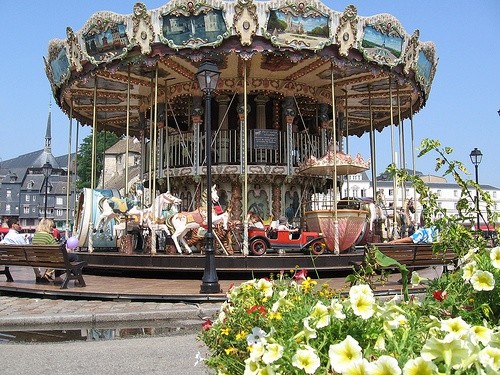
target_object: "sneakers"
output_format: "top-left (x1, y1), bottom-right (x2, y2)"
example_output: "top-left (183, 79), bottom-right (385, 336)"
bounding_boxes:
top-left (44, 274), bottom-right (54, 281)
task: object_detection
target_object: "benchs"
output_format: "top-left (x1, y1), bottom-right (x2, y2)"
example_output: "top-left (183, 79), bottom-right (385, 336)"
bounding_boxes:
top-left (348, 242), bottom-right (458, 286)
top-left (0, 243), bottom-right (88, 289)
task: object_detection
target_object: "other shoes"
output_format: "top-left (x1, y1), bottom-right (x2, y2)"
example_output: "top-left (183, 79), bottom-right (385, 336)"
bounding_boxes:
top-left (42, 277), bottom-right (48, 282)
top-left (36, 276), bottom-right (41, 281)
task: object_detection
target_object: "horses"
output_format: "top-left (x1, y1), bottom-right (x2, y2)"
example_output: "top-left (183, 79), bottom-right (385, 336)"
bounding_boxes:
top-left (94, 179), bottom-right (228, 255)
top-left (360, 189), bottom-right (416, 241)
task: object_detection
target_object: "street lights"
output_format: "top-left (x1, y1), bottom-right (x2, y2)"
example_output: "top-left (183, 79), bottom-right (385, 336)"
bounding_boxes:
top-left (41, 161), bottom-right (54, 219)
top-left (469, 147), bottom-right (484, 229)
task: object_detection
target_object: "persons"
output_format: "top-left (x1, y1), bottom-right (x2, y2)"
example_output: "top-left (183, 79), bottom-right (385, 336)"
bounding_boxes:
top-left (51, 225), bottom-right (60, 240)
top-left (277, 216), bottom-right (299, 240)
top-left (386, 226), bottom-right (438, 244)
top-left (1, 218), bottom-right (43, 282)
top-left (32, 219), bottom-right (67, 281)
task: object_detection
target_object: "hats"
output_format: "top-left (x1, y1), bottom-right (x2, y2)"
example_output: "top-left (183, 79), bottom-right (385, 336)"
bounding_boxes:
top-left (279, 216), bottom-right (288, 225)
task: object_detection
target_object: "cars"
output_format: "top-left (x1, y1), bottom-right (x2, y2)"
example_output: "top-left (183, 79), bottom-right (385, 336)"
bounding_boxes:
top-left (246, 220), bottom-right (328, 260)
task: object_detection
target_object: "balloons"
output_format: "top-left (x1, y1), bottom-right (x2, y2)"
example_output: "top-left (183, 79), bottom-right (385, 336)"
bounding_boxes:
top-left (67, 236), bottom-right (78, 249)
